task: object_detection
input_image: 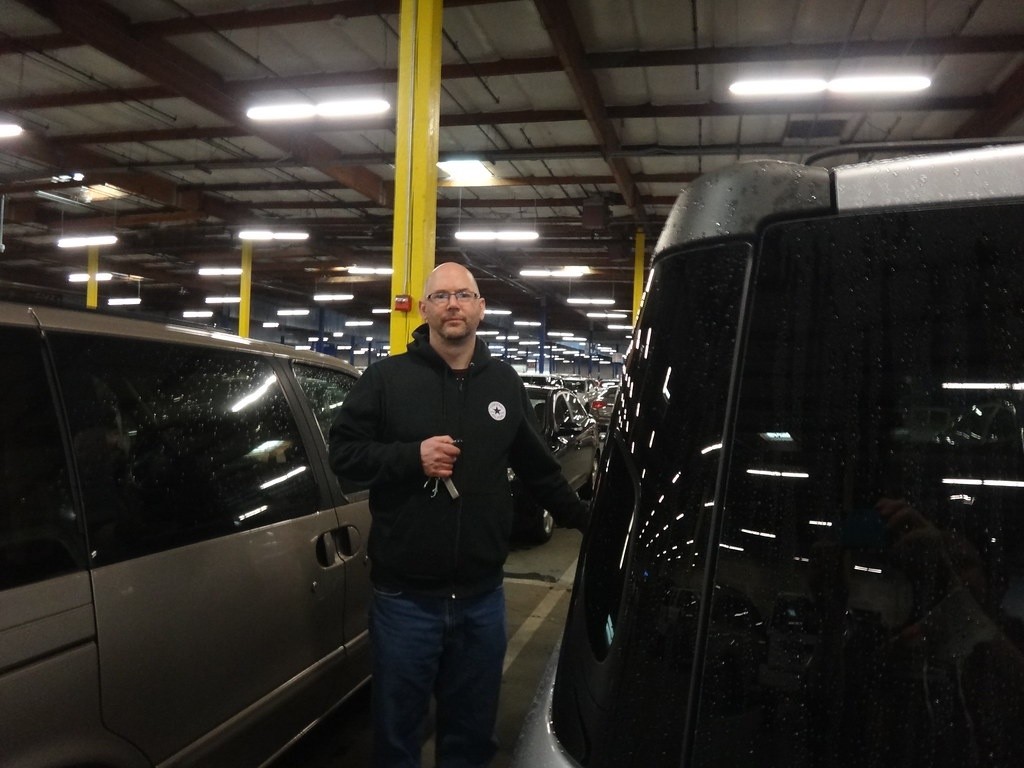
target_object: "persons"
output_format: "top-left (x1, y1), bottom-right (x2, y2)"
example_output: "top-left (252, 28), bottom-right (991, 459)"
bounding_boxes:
top-left (801, 453), bottom-right (1024, 768)
top-left (328, 262), bottom-right (588, 768)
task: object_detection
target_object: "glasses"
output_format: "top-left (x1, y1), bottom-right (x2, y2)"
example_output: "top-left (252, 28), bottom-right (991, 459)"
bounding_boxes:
top-left (426, 291), bottom-right (481, 303)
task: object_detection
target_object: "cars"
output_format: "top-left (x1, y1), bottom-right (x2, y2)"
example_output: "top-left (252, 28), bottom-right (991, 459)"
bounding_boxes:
top-left (0, 283), bottom-right (380, 768)
top-left (516, 369), bottom-right (622, 548)
top-left (547, 134), bottom-right (1024, 768)
